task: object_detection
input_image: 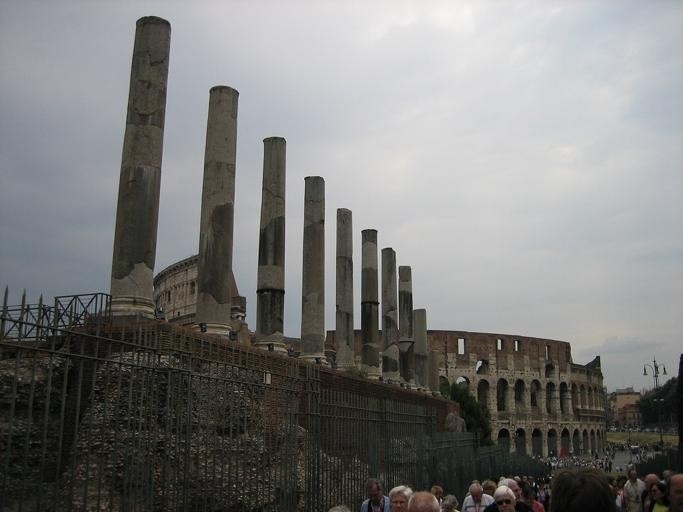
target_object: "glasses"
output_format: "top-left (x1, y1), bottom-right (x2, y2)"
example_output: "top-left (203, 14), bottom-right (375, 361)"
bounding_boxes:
top-left (495, 498), bottom-right (512, 505)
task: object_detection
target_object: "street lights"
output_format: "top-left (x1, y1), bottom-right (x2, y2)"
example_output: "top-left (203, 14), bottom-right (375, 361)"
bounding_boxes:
top-left (643, 355), bottom-right (667, 442)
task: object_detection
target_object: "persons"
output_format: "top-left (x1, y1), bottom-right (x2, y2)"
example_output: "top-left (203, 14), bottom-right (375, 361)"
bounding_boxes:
top-left (324, 439), bottom-right (683, 511)
